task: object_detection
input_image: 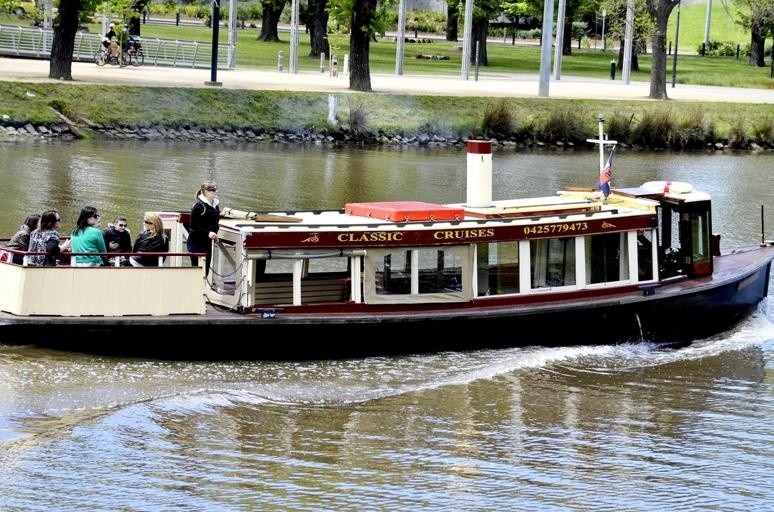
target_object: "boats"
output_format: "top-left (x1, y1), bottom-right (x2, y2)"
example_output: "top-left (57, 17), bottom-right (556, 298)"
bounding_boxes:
top-left (0, 116), bottom-right (774, 366)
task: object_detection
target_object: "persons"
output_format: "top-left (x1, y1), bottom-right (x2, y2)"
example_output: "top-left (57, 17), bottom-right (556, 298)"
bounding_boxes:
top-left (69, 206), bottom-right (107, 268)
top-left (101, 215), bottom-right (133, 267)
top-left (120, 210), bottom-right (169, 268)
top-left (101, 22), bottom-right (137, 64)
top-left (27, 210), bottom-right (71, 267)
top-left (187, 181), bottom-right (220, 266)
top-left (0, 214), bottom-right (41, 265)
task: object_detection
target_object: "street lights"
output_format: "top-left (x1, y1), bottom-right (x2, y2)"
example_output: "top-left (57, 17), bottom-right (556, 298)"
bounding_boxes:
top-left (601, 10), bottom-right (607, 53)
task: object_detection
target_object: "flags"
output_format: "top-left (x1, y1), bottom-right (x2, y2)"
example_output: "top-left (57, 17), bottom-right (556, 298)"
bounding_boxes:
top-left (593, 145), bottom-right (613, 198)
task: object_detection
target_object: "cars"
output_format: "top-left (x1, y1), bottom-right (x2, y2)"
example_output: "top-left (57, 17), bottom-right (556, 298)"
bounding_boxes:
top-left (2, 0), bottom-right (37, 16)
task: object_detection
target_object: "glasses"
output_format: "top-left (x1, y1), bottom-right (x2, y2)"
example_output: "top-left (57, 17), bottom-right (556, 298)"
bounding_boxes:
top-left (56, 219), bottom-right (61, 222)
top-left (119, 224), bottom-right (127, 227)
top-left (145, 221), bottom-right (153, 224)
top-left (207, 188), bottom-right (217, 192)
top-left (91, 215), bottom-right (99, 219)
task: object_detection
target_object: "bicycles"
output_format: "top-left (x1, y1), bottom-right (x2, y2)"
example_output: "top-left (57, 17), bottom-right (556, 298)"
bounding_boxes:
top-left (116, 40), bottom-right (143, 66)
top-left (93, 42), bottom-right (116, 66)
